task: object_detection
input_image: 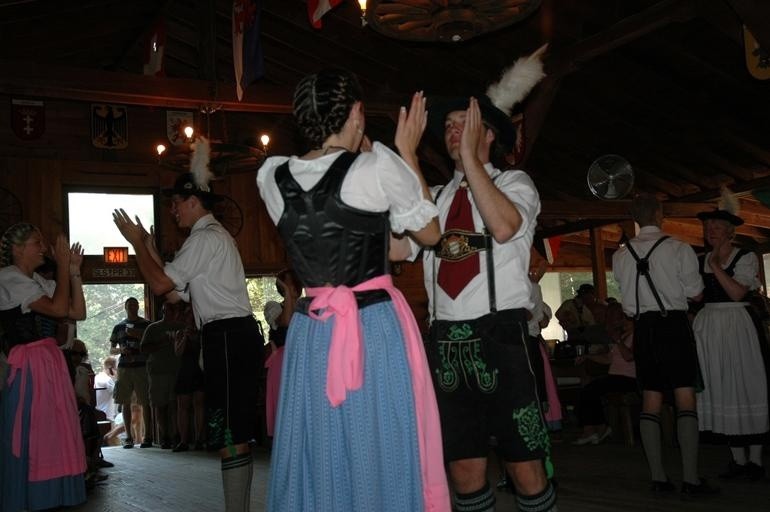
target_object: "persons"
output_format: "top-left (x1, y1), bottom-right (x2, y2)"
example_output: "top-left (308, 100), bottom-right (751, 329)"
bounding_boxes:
top-left (113, 173), bottom-right (265, 512)
top-left (65, 339), bottom-right (109, 483)
top-left (693, 184), bottom-right (770, 479)
top-left (110, 298), bottom-right (153, 449)
top-left (140, 302), bottom-right (180, 449)
top-left (257, 77), bottom-right (451, 512)
top-left (556, 284), bottom-right (610, 351)
top-left (389, 94), bottom-right (558, 512)
top-left (173, 306), bottom-right (205, 452)
top-left (263, 270), bottom-right (303, 436)
top-left (575, 314), bottom-right (637, 447)
top-left (70, 274), bottom-right (82, 278)
top-left (528, 247), bottom-right (562, 421)
top-left (94, 356), bottom-right (118, 425)
top-left (0, 224), bottom-right (87, 512)
top-left (567, 303), bottom-right (611, 447)
top-left (102, 380), bottom-right (126, 445)
top-left (613, 194), bottom-right (722, 499)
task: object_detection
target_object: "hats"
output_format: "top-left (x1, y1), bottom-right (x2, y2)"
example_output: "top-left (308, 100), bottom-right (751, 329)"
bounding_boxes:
top-left (162, 136), bottom-right (223, 202)
top-left (426, 43), bottom-right (552, 156)
top-left (697, 185), bottom-right (744, 226)
top-left (576, 284), bottom-right (596, 293)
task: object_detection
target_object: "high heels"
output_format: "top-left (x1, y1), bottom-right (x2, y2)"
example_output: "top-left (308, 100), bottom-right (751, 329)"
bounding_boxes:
top-left (574, 434), bottom-right (598, 445)
top-left (599, 426), bottom-right (612, 442)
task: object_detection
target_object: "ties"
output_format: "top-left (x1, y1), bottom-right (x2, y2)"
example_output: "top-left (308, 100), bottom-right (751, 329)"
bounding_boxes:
top-left (436, 176), bottom-right (481, 300)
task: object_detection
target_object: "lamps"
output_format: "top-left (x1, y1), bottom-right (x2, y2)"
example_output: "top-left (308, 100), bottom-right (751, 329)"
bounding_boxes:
top-left (356, 0), bottom-right (544, 44)
top-left (148, 100), bottom-right (271, 175)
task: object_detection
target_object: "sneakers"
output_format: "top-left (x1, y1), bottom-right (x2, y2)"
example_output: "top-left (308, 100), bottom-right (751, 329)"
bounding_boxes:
top-left (124, 440), bottom-right (133, 448)
top-left (141, 439), bottom-right (152, 447)
top-left (173, 443), bottom-right (189, 451)
top-left (160, 441), bottom-right (171, 448)
top-left (195, 442), bottom-right (202, 450)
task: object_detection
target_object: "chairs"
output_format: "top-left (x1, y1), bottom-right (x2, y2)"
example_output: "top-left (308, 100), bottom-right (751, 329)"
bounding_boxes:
top-left (604, 387), bottom-right (673, 447)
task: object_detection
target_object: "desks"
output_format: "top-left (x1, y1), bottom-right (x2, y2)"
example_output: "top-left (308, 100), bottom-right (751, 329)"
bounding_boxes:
top-left (543, 341), bottom-right (607, 426)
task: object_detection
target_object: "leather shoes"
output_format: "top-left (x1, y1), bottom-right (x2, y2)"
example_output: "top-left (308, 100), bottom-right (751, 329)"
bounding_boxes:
top-left (737, 462), bottom-right (765, 481)
top-left (719, 463), bottom-right (745, 480)
top-left (680, 482), bottom-right (721, 500)
top-left (652, 479), bottom-right (680, 497)
